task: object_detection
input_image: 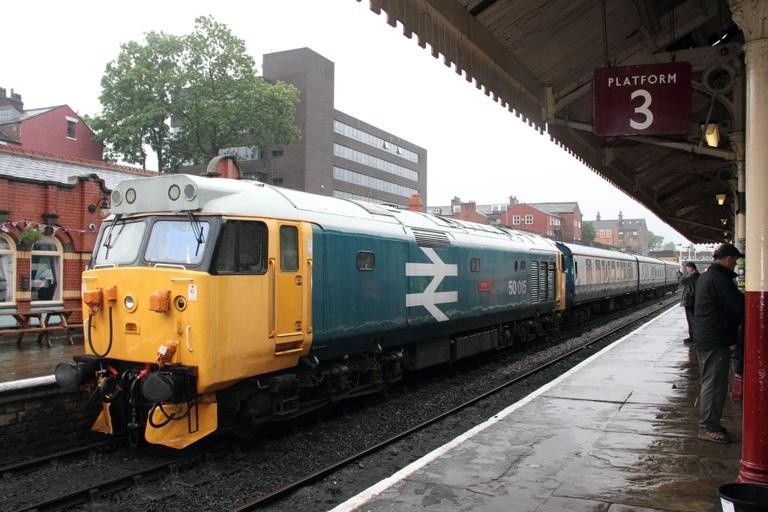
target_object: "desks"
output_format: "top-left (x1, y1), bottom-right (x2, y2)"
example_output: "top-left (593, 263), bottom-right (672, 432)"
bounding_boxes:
top-left (1, 308), bottom-right (82, 348)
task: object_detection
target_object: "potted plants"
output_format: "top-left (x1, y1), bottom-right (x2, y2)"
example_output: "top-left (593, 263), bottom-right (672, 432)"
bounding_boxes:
top-left (18, 230), bottom-right (42, 248)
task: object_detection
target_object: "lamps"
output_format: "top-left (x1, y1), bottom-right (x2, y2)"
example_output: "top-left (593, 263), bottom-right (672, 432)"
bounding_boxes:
top-left (715, 191), bottom-right (726, 207)
top-left (698, 121), bottom-right (722, 147)
top-left (88, 194), bottom-right (112, 219)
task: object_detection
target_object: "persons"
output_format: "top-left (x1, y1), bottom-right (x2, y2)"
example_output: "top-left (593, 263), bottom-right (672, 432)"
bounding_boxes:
top-left (689, 241), bottom-right (744, 447)
top-left (674, 260), bottom-right (700, 344)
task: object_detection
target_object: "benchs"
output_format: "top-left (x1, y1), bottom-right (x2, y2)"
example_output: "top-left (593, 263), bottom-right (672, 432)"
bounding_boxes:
top-left (0, 324), bottom-right (83, 348)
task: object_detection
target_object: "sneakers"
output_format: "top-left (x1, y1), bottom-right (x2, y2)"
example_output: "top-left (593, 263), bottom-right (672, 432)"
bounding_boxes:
top-left (719, 425), bottom-right (728, 435)
top-left (696, 430), bottom-right (729, 444)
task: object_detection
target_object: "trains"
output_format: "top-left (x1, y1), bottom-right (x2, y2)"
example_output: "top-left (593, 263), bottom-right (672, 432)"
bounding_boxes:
top-left (52, 151), bottom-right (685, 453)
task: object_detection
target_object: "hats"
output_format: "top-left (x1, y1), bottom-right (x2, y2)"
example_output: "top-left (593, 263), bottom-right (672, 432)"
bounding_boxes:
top-left (713, 242), bottom-right (744, 258)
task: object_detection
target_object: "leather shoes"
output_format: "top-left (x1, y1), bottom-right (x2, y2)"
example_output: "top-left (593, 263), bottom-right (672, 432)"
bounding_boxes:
top-left (683, 338), bottom-right (693, 343)
